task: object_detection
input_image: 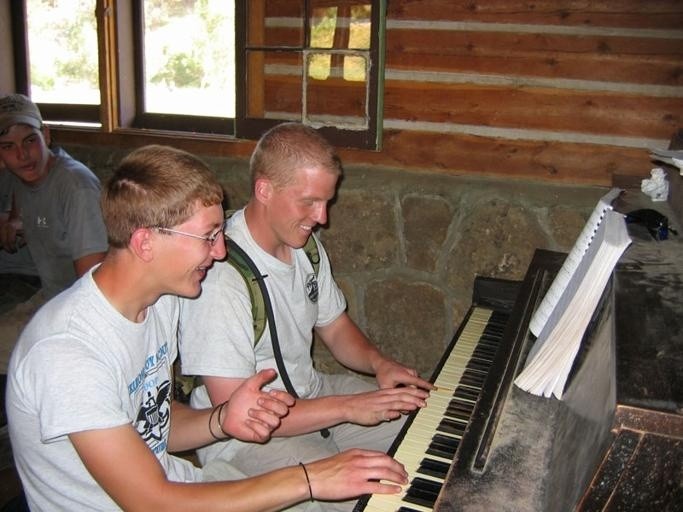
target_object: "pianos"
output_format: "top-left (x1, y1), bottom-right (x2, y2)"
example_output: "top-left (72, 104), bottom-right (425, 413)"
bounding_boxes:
top-left (353, 165), bottom-right (681, 508)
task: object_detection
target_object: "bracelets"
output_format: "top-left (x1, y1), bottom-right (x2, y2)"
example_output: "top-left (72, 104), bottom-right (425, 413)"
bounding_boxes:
top-left (297, 460), bottom-right (314, 504)
top-left (207, 400), bottom-right (232, 444)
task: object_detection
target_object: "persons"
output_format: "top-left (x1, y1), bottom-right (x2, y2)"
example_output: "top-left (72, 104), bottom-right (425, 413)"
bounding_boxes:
top-left (0, 92), bottom-right (110, 372)
top-left (3, 143), bottom-right (409, 510)
top-left (176, 121), bottom-right (436, 478)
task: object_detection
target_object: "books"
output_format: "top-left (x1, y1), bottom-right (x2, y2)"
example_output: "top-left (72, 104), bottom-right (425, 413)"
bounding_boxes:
top-left (515, 186), bottom-right (633, 400)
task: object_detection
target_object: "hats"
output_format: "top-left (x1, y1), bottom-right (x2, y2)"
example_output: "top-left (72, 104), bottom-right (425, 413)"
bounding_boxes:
top-left (0, 93), bottom-right (43, 131)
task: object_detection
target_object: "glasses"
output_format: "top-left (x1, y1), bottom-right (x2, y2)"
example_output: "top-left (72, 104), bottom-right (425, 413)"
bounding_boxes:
top-left (158, 223), bottom-right (226, 245)
